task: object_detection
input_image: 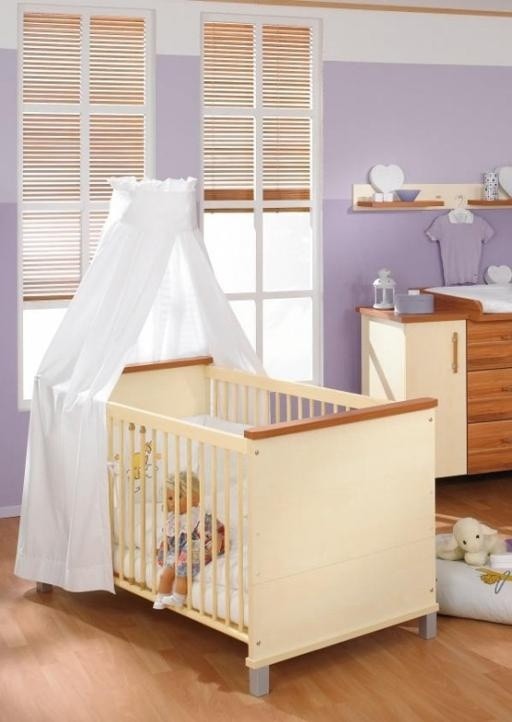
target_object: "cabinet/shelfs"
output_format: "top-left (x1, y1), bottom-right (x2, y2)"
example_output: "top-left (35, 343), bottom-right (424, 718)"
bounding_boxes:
top-left (358, 283), bottom-right (512, 478)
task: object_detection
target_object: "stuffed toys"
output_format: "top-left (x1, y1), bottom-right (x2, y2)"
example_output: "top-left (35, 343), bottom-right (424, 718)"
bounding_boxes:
top-left (435, 517), bottom-right (506, 566)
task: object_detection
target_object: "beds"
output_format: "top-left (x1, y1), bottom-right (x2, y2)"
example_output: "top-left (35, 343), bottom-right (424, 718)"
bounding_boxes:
top-left (47, 356), bottom-right (439, 698)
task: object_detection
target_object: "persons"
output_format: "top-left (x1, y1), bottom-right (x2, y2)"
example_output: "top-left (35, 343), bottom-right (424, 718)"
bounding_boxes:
top-left (153, 469), bottom-right (225, 609)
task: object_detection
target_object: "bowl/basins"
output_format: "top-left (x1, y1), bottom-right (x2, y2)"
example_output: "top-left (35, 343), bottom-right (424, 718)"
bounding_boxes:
top-left (394, 189), bottom-right (422, 202)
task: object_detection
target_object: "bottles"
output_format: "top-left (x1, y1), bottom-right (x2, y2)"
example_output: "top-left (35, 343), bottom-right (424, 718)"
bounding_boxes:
top-left (481, 168), bottom-right (499, 200)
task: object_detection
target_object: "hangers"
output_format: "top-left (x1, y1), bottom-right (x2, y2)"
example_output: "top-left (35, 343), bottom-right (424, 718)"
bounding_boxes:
top-left (453, 195), bottom-right (468, 221)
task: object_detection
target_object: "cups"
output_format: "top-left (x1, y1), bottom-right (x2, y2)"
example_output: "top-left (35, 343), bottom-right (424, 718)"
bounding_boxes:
top-left (373, 192), bottom-right (394, 202)
